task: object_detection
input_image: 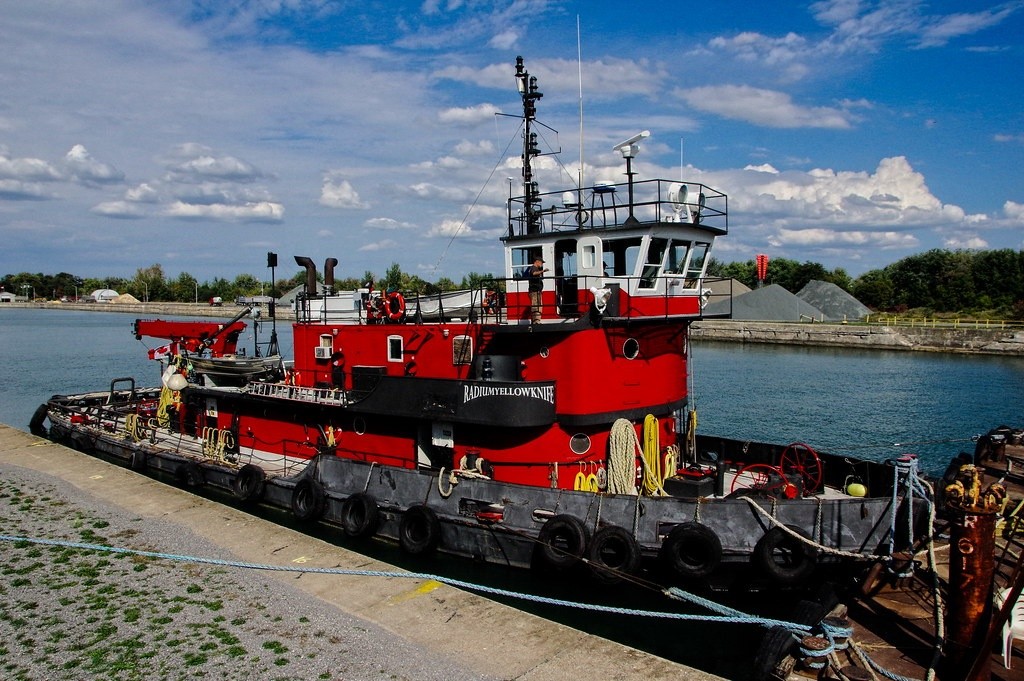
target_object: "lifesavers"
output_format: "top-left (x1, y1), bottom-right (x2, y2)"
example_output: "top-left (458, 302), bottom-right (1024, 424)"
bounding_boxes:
top-left (386, 292), bottom-right (404, 319)
top-left (484, 291), bottom-right (499, 314)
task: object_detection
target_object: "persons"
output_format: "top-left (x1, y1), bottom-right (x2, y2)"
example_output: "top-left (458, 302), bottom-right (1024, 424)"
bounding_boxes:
top-left (526, 256), bottom-right (549, 324)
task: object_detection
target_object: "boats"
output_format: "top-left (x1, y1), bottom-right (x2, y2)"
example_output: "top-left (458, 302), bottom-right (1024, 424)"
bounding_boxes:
top-left (39, 56), bottom-right (955, 681)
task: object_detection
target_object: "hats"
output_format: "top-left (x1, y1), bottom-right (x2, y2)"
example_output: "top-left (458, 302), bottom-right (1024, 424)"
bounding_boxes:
top-left (533, 256), bottom-right (545, 263)
